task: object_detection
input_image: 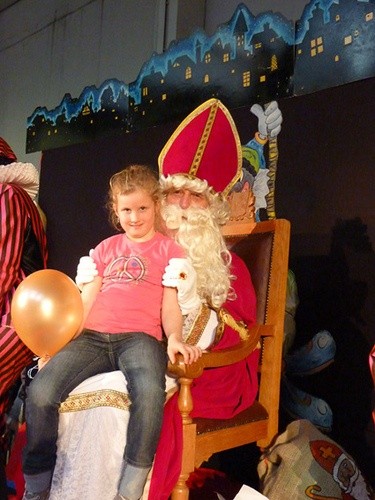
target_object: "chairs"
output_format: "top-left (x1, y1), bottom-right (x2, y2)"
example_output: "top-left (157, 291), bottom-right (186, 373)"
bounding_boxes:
top-left (166, 218), bottom-right (292, 500)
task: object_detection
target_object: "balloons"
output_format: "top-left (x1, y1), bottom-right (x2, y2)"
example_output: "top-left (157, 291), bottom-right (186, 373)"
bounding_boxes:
top-left (11, 269), bottom-right (82, 361)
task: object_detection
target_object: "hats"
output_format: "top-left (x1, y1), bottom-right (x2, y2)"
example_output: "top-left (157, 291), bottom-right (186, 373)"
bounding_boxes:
top-left (158, 99), bottom-right (243, 196)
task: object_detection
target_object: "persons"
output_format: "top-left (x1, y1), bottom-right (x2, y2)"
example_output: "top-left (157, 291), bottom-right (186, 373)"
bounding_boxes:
top-left (0, 138), bottom-right (47, 459)
top-left (21, 167), bottom-right (201, 499)
top-left (8, 98), bottom-right (260, 500)
